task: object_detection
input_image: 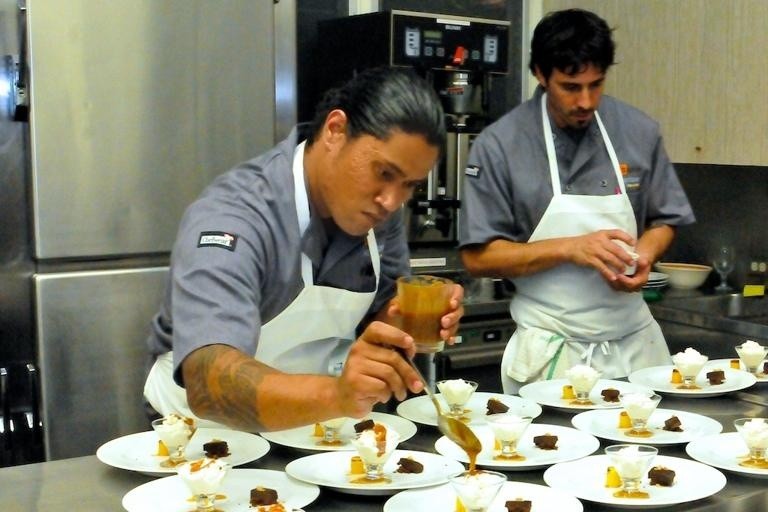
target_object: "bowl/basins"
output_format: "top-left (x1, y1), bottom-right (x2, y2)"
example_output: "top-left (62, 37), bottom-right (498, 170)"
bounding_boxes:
top-left (653, 263), bottom-right (712, 290)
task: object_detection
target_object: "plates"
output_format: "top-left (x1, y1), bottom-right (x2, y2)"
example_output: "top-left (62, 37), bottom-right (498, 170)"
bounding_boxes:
top-left (434, 424), bottom-right (599, 472)
top-left (121, 467), bottom-right (320, 512)
top-left (380, 480), bottom-right (582, 512)
top-left (96, 429), bottom-right (271, 478)
top-left (627, 364), bottom-right (757, 399)
top-left (517, 378), bottom-right (655, 415)
top-left (570, 409), bottom-right (723, 448)
top-left (259, 411), bottom-right (417, 454)
top-left (685, 431), bottom-right (768, 480)
top-left (640, 271), bottom-right (669, 289)
top-left (284, 451), bottom-right (466, 497)
top-left (706, 357), bottom-right (766, 386)
top-left (396, 391), bottom-right (542, 428)
top-left (541, 453), bottom-right (727, 510)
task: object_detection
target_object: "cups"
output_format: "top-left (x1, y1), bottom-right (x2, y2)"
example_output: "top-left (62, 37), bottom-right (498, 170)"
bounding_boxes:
top-left (397, 275), bottom-right (453, 354)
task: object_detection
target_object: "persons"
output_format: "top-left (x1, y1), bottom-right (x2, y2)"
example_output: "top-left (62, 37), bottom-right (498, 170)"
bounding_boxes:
top-left (455, 7), bottom-right (700, 400)
top-left (140, 65), bottom-right (468, 436)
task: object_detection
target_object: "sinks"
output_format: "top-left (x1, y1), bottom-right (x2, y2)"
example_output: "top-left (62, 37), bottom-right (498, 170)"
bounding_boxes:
top-left (655, 276), bottom-right (768, 342)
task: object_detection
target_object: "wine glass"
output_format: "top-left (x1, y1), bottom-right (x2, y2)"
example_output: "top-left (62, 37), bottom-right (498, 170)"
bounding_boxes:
top-left (445, 469), bottom-right (506, 512)
top-left (603, 444), bottom-right (658, 497)
top-left (732, 418), bottom-right (767, 469)
top-left (318, 414), bottom-right (345, 445)
top-left (174, 462), bottom-right (229, 512)
top-left (564, 370), bottom-right (602, 405)
top-left (711, 244), bottom-right (735, 291)
top-left (617, 393), bottom-right (661, 437)
top-left (734, 345), bottom-right (767, 377)
top-left (436, 380), bottom-right (478, 417)
top-left (152, 418), bottom-right (198, 468)
top-left (485, 415), bottom-right (531, 458)
top-left (349, 433), bottom-right (398, 479)
top-left (671, 354), bottom-right (707, 390)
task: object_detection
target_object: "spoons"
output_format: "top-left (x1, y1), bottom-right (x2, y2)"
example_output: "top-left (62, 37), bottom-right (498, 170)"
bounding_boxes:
top-left (390, 345), bottom-right (481, 453)
